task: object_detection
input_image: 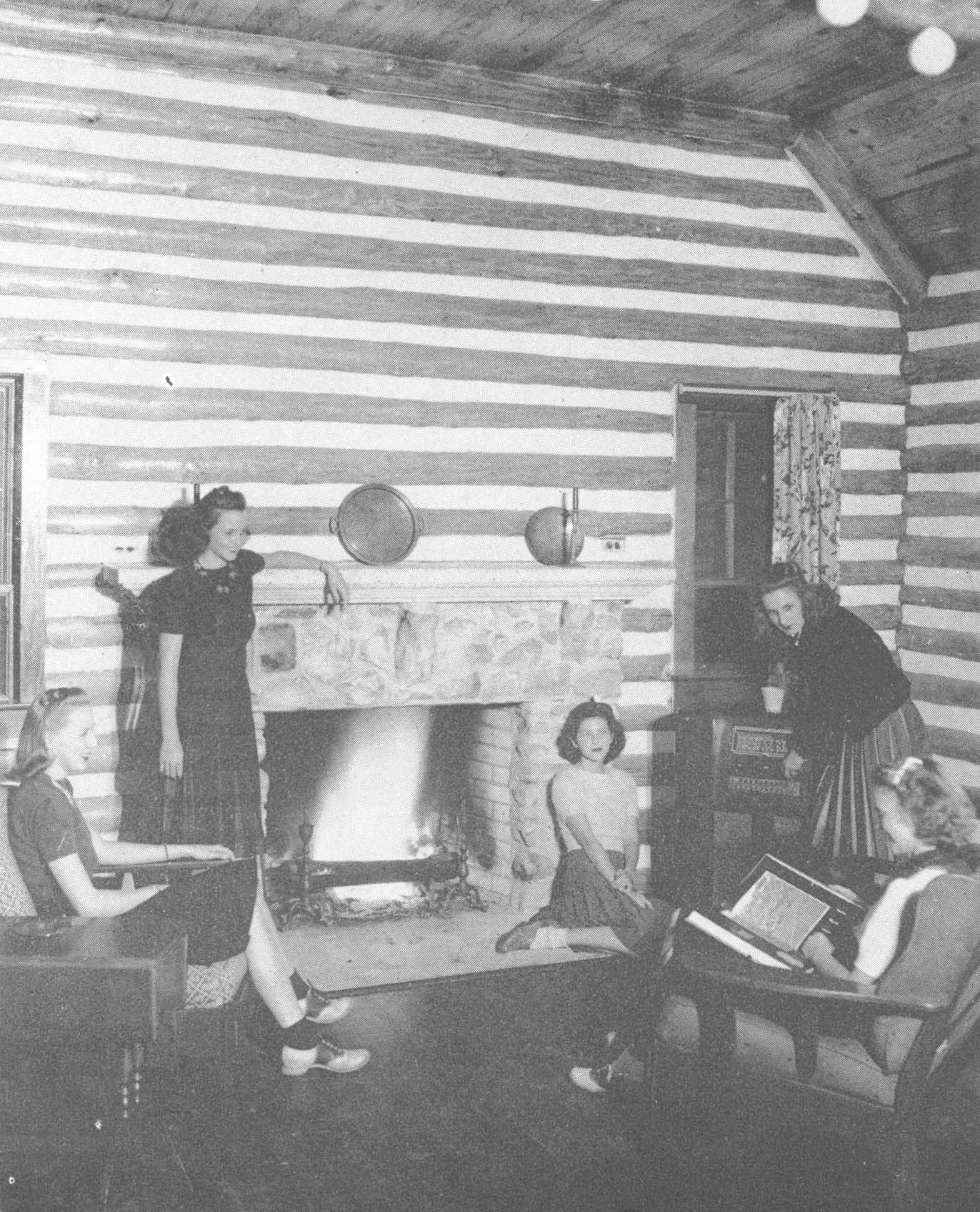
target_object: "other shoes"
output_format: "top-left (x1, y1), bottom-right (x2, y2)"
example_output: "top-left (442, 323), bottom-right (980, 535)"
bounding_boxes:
top-left (571, 1065), bottom-right (648, 1096)
top-left (498, 915), bottom-right (555, 953)
top-left (281, 1038), bottom-right (371, 1076)
top-left (296, 985), bottom-right (352, 1023)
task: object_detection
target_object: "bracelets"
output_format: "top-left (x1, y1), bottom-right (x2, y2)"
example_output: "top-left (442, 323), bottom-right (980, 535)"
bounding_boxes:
top-left (163, 843), bottom-right (169, 861)
top-left (319, 560), bottom-right (328, 572)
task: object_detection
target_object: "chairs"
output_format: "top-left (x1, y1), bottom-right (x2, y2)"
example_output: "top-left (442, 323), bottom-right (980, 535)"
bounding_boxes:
top-left (626, 853), bottom-right (980, 1210)
top-left (4, 770), bottom-right (265, 1083)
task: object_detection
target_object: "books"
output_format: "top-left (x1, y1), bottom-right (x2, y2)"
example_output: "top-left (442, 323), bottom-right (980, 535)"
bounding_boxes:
top-left (684, 852), bottom-right (869, 977)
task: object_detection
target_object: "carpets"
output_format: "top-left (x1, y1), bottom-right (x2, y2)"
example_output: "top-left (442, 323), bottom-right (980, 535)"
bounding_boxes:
top-left (280, 902), bottom-right (628, 998)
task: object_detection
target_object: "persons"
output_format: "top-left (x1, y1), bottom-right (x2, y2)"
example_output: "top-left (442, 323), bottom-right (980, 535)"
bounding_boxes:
top-left (1, 687), bottom-right (371, 1077)
top-left (495, 695), bottom-right (676, 961)
top-left (753, 562), bottom-right (932, 864)
top-left (569, 753), bottom-right (980, 1095)
top-left (129, 486), bottom-right (349, 859)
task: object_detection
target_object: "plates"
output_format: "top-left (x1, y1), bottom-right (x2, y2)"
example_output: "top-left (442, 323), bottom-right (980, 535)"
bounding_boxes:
top-left (11, 916), bottom-right (71, 950)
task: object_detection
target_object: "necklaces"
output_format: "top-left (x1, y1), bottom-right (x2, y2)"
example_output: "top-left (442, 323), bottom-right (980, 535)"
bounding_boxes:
top-left (192, 557), bottom-right (238, 595)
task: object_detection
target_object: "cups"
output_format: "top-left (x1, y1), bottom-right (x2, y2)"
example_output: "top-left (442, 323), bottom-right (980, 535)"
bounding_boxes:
top-left (762, 686), bottom-right (784, 714)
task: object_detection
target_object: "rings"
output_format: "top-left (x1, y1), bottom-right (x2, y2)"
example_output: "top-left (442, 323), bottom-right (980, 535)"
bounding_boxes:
top-left (624, 885), bottom-right (628, 890)
